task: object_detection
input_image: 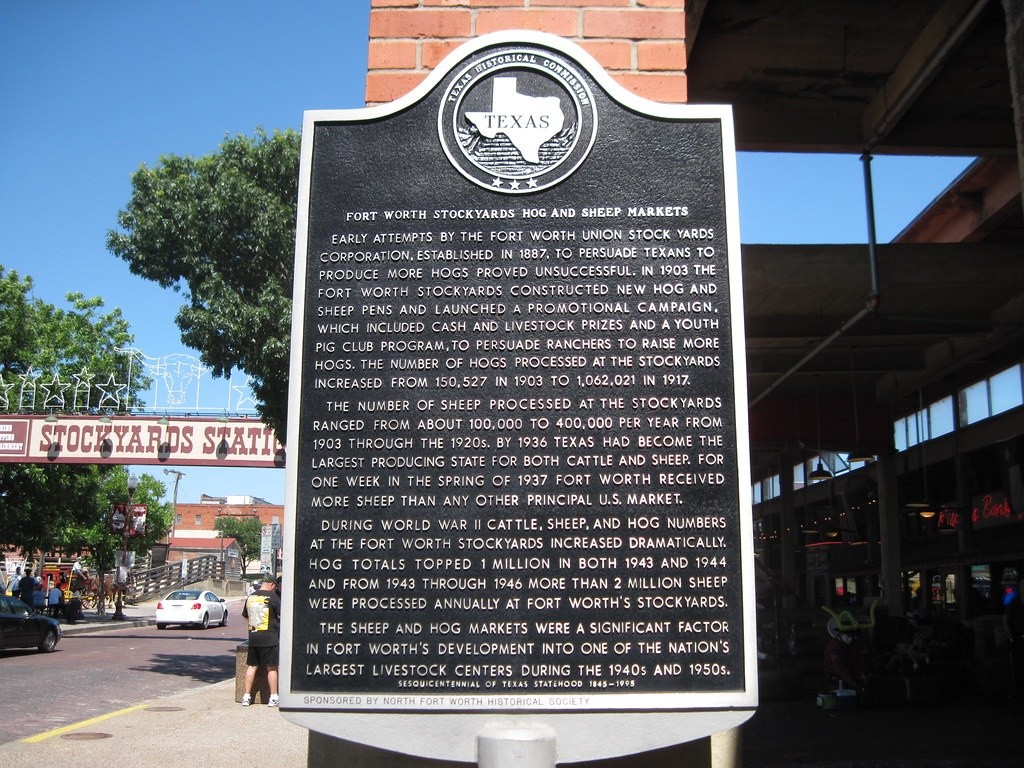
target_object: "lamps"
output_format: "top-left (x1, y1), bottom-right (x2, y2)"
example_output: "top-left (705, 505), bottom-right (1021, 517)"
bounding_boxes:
top-left (99, 408), bottom-right (113, 423)
top-left (45, 408), bottom-right (58, 422)
top-left (899, 387), bottom-right (968, 517)
top-left (846, 345), bottom-right (874, 461)
top-left (157, 410), bottom-right (170, 425)
top-left (800, 479), bottom-right (858, 537)
top-left (808, 388), bottom-right (832, 479)
top-left (216, 408), bottom-right (229, 422)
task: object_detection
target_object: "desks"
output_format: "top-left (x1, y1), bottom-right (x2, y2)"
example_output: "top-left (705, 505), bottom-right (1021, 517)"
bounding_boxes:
top-left (43, 596), bottom-right (69, 613)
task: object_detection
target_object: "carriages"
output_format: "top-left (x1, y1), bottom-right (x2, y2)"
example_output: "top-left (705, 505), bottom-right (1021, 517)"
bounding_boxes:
top-left (39, 564), bottom-right (131, 609)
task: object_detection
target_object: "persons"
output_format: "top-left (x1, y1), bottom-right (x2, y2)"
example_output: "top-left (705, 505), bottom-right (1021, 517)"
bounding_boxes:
top-left (0, 556), bottom-right (87, 623)
top-left (821, 621), bottom-right (876, 696)
top-left (242, 572), bottom-right (281, 707)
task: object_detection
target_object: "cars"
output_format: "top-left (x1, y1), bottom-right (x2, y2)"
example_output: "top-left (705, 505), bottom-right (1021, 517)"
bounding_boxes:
top-left (155, 589), bottom-right (229, 629)
top-left (0, 595), bottom-right (62, 653)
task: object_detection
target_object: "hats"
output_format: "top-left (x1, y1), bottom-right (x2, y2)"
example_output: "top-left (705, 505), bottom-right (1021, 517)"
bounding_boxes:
top-left (71, 591), bottom-right (81, 598)
top-left (262, 573), bottom-right (278, 586)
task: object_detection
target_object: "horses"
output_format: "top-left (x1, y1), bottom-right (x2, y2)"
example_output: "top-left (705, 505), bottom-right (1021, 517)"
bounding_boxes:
top-left (88, 575), bottom-right (130, 609)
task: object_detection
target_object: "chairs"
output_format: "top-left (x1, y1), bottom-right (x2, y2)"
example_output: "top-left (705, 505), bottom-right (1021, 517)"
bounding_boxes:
top-left (179, 595), bottom-right (186, 600)
